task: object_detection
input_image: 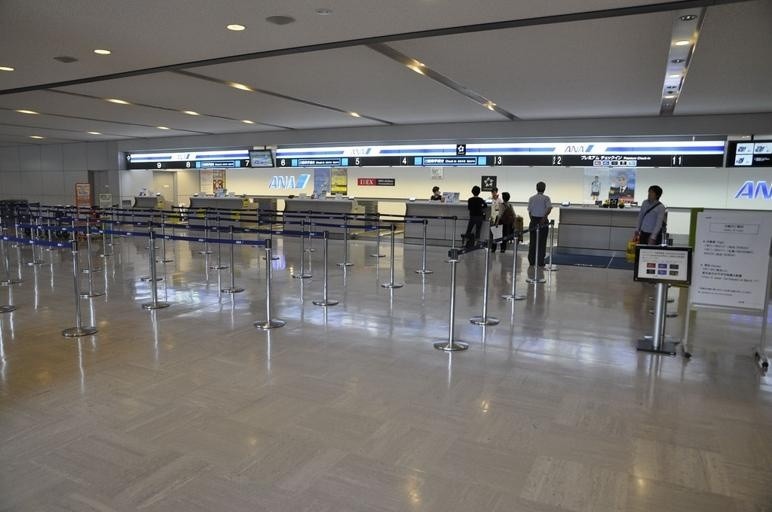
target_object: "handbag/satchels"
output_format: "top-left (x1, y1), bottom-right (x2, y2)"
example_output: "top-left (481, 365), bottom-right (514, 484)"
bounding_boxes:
top-left (625, 241), bottom-right (636, 263)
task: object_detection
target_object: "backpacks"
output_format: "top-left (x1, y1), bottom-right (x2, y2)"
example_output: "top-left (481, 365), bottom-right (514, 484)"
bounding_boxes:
top-left (502, 203), bottom-right (515, 226)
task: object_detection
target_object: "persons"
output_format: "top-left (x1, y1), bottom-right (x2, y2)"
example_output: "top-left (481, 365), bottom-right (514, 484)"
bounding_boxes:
top-left (431, 186), bottom-right (441, 201)
top-left (633, 184), bottom-right (666, 245)
top-left (464, 185), bottom-right (487, 239)
top-left (490, 192), bottom-right (514, 254)
top-left (527, 181), bottom-right (552, 266)
top-left (611, 174), bottom-right (633, 199)
top-left (487, 187), bottom-right (503, 221)
top-left (590, 176), bottom-right (601, 203)
